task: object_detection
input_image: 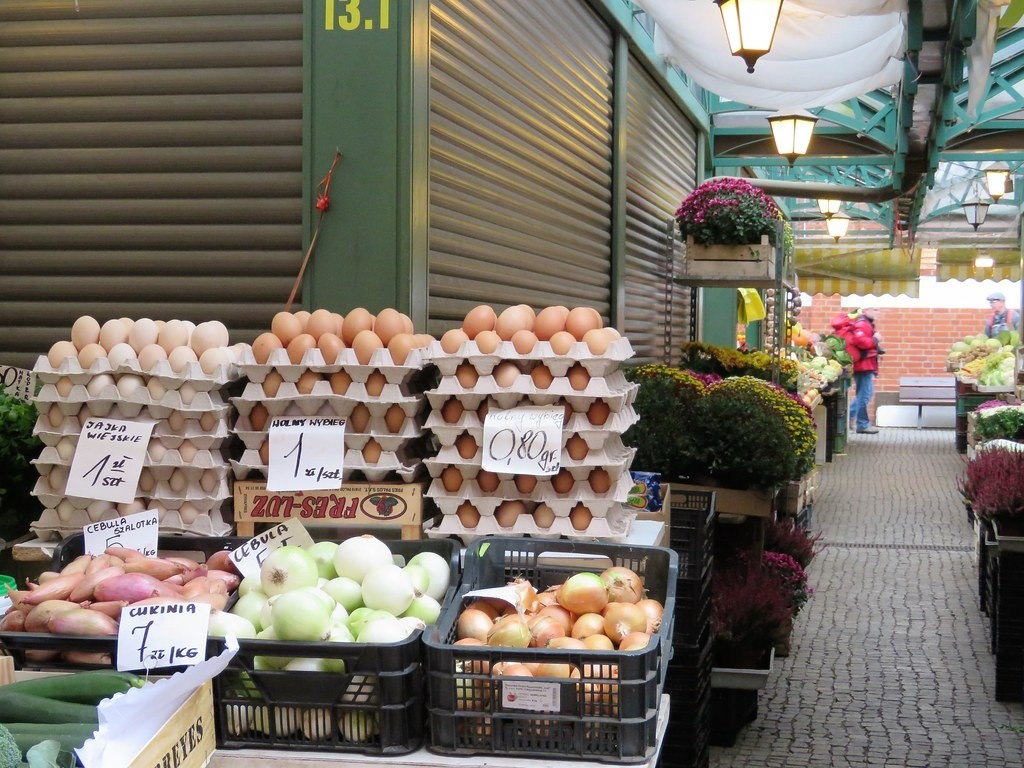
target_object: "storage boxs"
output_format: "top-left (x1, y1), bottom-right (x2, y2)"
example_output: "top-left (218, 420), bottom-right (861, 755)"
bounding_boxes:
top-left (0, 300), bottom-right (718, 766)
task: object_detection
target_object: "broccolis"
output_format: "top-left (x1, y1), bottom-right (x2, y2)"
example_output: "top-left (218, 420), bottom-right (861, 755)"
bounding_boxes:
top-left (0, 723), bottom-right (23, 768)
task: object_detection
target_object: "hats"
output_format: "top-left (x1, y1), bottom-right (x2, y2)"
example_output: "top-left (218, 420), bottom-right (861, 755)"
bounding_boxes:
top-left (986, 294), bottom-right (1005, 301)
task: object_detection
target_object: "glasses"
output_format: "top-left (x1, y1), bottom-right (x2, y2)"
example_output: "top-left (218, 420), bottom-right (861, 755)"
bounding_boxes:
top-left (989, 300), bottom-right (998, 302)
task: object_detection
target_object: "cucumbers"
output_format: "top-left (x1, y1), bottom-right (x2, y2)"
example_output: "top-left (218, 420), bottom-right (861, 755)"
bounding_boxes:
top-left (0, 670), bottom-right (144, 755)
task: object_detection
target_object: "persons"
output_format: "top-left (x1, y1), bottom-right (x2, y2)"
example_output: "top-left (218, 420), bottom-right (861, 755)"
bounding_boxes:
top-left (831, 306), bottom-right (885, 435)
top-left (984, 294), bottom-right (1021, 338)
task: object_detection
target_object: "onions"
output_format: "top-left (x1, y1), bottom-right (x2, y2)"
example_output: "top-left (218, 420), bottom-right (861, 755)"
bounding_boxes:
top-left (0, 547), bottom-right (241, 665)
top-left (206, 533), bottom-right (451, 741)
top-left (453, 567), bottom-right (664, 744)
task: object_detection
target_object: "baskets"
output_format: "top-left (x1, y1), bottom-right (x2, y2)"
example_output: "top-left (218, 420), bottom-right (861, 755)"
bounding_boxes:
top-left (658, 488), bottom-right (718, 768)
top-left (821, 374), bottom-right (849, 463)
top-left (423, 535), bottom-right (680, 766)
top-left (1, 530), bottom-right (462, 758)
top-left (954, 375), bottom-right (998, 454)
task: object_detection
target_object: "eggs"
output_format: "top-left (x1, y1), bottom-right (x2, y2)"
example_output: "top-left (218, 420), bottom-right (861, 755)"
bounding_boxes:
top-left (48, 315), bottom-right (249, 524)
top-left (441, 304), bottom-right (622, 530)
top-left (249, 307), bottom-right (436, 465)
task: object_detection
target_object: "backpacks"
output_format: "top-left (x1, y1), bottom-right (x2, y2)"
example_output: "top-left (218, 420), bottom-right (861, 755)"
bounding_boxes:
top-left (830, 310), bottom-right (875, 364)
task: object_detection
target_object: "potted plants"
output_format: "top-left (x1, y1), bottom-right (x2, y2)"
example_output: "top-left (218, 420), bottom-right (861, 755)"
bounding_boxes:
top-left (614, 332), bottom-right (825, 663)
top-left (962, 405), bottom-right (1024, 530)
top-left (680, 178), bottom-right (795, 251)
